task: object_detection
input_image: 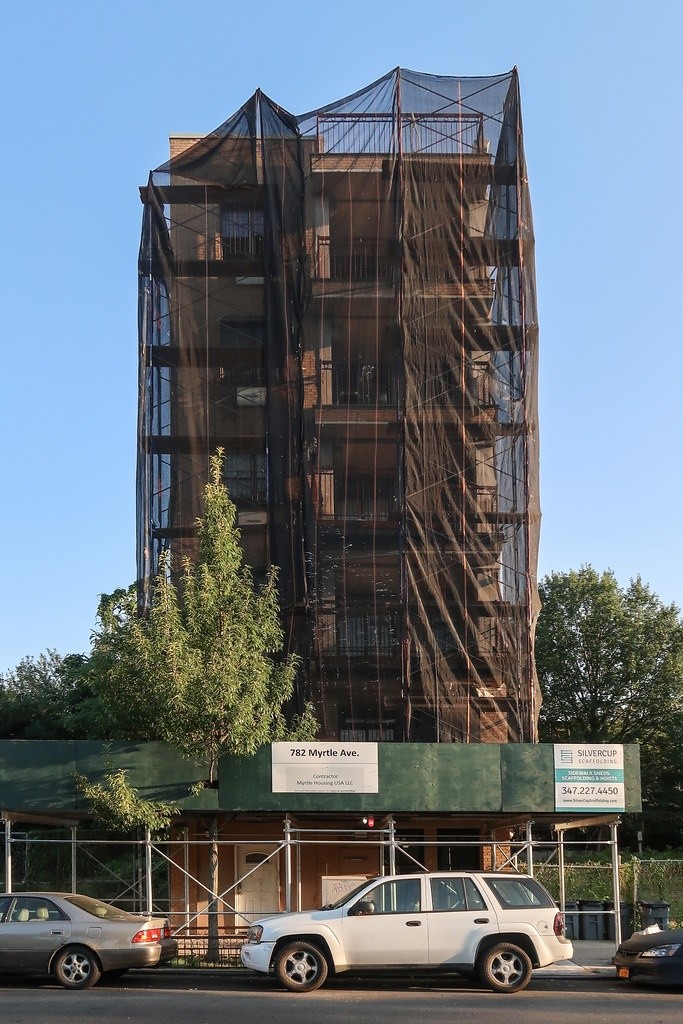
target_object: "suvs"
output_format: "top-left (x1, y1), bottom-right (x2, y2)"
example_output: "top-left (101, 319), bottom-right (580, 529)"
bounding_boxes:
top-left (241, 870), bottom-right (575, 994)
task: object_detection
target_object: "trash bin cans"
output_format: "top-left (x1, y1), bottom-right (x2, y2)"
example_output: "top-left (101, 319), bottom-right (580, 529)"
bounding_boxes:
top-left (556, 901), bottom-right (579, 941)
top-left (636, 899), bottom-right (670, 931)
top-left (603, 900), bottom-right (631, 941)
top-left (578, 900), bottom-right (604, 940)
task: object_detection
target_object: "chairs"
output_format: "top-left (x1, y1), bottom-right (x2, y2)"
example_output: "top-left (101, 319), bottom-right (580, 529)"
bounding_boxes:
top-left (29, 907), bottom-right (49, 923)
top-left (10, 907), bottom-right (30, 922)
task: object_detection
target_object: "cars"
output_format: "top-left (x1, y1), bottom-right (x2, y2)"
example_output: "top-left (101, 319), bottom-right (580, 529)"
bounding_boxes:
top-left (612, 928), bottom-right (683, 986)
top-left (0, 892), bottom-right (179, 989)
